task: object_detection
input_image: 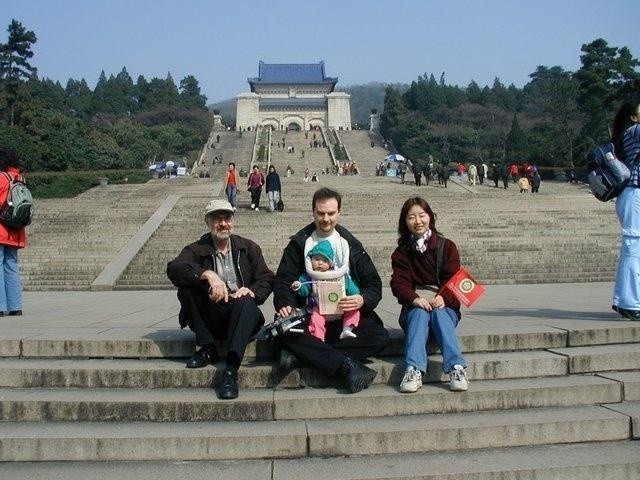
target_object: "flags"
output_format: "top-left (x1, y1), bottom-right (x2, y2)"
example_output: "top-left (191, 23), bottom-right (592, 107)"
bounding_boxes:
top-left (446, 267), bottom-right (486, 308)
top-left (317, 281), bottom-right (344, 315)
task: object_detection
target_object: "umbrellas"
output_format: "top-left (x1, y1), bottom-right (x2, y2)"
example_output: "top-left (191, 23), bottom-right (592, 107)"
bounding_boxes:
top-left (383, 153), bottom-right (407, 162)
top-left (156, 158), bottom-right (185, 179)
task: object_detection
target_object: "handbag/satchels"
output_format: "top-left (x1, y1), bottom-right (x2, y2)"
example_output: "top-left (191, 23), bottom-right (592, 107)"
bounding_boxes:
top-left (277, 200), bottom-right (284, 211)
top-left (415, 284), bottom-right (441, 304)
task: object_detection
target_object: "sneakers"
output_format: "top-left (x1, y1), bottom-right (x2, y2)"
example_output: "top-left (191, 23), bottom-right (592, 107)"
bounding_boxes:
top-left (612, 305), bottom-right (630, 319)
top-left (448, 364), bottom-right (470, 391)
top-left (618, 305), bottom-right (640, 321)
top-left (233, 204), bottom-right (277, 212)
top-left (399, 365), bottom-right (423, 393)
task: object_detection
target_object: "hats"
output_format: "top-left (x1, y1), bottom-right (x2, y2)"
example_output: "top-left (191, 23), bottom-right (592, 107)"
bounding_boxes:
top-left (204, 199), bottom-right (235, 216)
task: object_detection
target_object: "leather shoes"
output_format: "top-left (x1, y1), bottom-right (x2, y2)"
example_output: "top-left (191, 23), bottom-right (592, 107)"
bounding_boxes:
top-left (339, 356), bottom-right (377, 393)
top-left (187, 345), bottom-right (218, 367)
top-left (9, 310), bottom-right (22, 315)
top-left (220, 370), bottom-right (238, 398)
top-left (277, 349), bottom-right (303, 377)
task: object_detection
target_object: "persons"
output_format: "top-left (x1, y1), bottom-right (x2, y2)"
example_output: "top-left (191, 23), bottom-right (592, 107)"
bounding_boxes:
top-left (199, 134), bottom-right (223, 178)
top-left (289, 239), bottom-right (361, 342)
top-left (609, 89), bottom-right (640, 321)
top-left (224, 162), bottom-right (240, 210)
top-left (371, 141), bottom-right (374, 148)
top-left (0, 144), bottom-right (28, 317)
top-left (265, 165), bottom-right (281, 211)
top-left (376, 161), bottom-right (450, 188)
top-left (277, 126), bottom-right (326, 158)
top-left (390, 197), bottom-right (470, 392)
top-left (338, 126), bottom-right (361, 130)
top-left (303, 160), bottom-right (360, 183)
top-left (457, 161), bottom-right (541, 192)
top-left (227, 122), bottom-right (260, 138)
top-left (286, 164), bottom-right (294, 177)
top-left (165, 199), bottom-right (275, 400)
top-left (247, 164), bottom-right (265, 211)
top-left (272, 186), bottom-right (389, 393)
top-left (569, 170), bottom-right (578, 183)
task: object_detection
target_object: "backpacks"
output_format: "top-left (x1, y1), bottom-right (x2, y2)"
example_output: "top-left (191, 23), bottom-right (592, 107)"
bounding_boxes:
top-left (585, 138), bottom-right (639, 202)
top-left (0, 170), bottom-right (33, 229)
